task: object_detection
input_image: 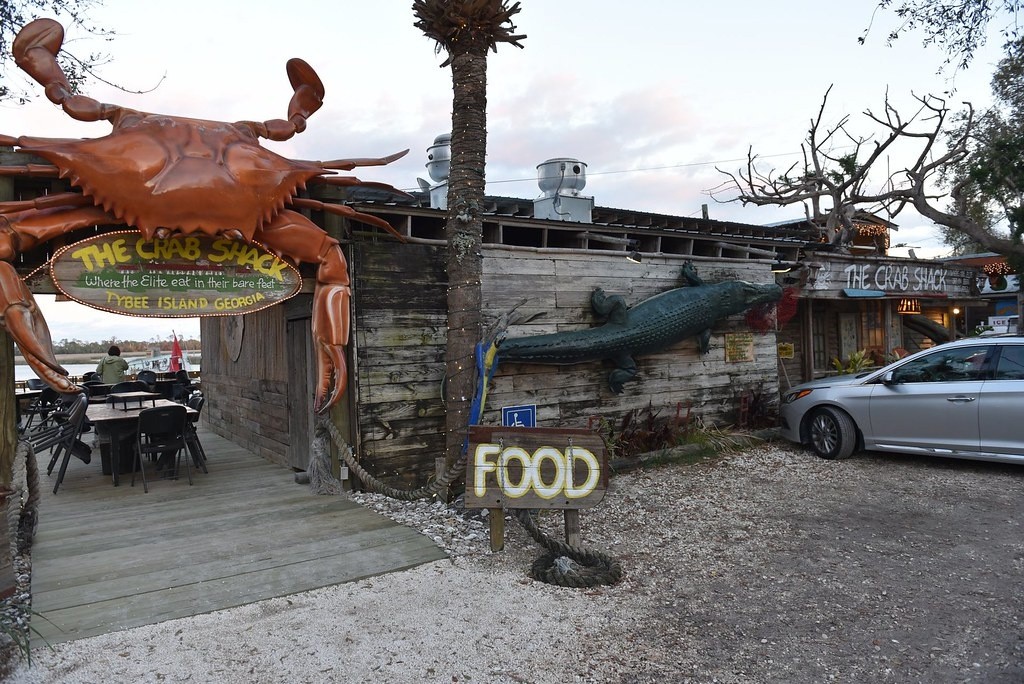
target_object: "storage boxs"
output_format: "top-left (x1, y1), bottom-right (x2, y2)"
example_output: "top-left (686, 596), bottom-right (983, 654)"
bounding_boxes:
top-left (532, 157), bottom-right (594, 223)
top-left (424, 133), bottom-right (451, 211)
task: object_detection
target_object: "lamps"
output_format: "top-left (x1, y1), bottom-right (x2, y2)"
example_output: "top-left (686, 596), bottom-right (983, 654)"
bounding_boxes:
top-left (897, 298), bottom-right (921, 315)
top-left (953, 307), bottom-right (960, 314)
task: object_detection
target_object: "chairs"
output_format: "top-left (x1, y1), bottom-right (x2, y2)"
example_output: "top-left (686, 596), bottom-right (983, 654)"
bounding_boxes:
top-left (15, 371), bottom-right (208, 494)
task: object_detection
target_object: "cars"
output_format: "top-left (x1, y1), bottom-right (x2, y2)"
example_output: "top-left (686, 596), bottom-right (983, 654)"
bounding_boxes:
top-left (779, 335), bottom-right (1024, 466)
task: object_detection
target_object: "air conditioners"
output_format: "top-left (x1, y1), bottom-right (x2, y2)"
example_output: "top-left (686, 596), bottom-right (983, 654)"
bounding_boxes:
top-left (988, 315), bottom-right (1019, 333)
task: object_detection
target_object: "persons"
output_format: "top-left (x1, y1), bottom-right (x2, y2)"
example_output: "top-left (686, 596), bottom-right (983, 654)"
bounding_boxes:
top-left (96, 345), bottom-right (129, 384)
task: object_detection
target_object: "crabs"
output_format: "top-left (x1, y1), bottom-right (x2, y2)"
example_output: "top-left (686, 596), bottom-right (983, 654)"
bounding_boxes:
top-left (0, 18), bottom-right (417, 416)
